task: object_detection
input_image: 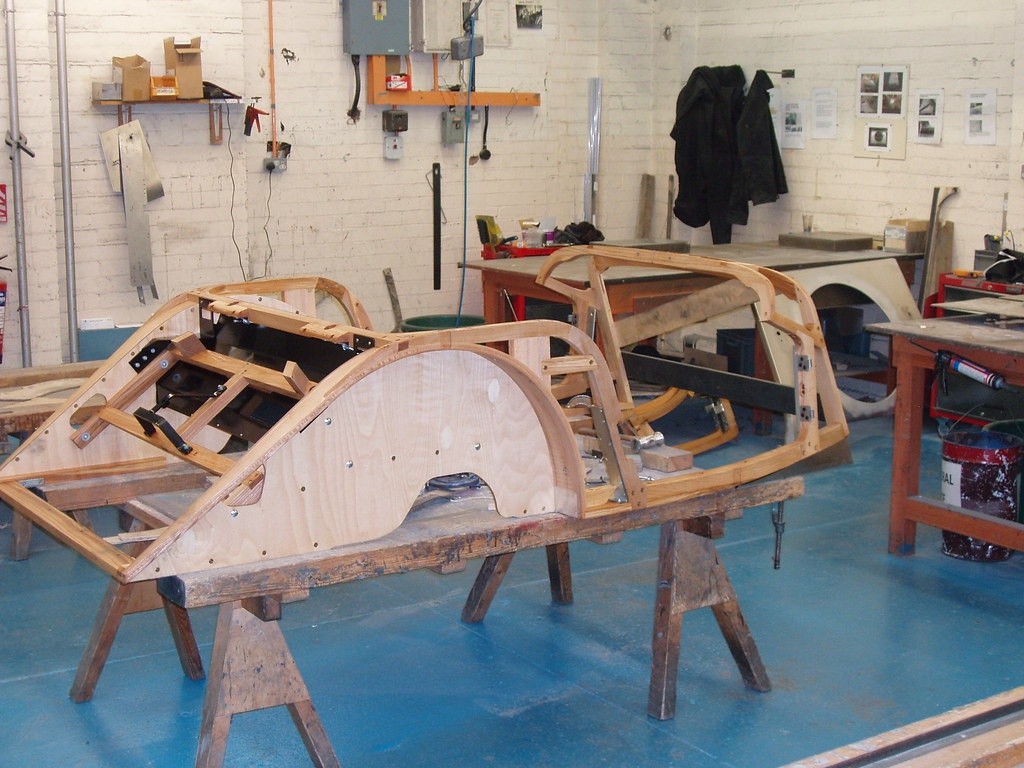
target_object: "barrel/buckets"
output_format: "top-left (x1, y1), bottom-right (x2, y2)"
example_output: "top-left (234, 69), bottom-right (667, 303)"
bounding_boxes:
top-left (941, 402), bottom-right (1024, 562)
top-left (400, 313), bottom-right (485, 346)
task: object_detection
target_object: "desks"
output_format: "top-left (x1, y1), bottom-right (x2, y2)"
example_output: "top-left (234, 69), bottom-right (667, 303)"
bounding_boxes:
top-left (867, 314), bottom-right (1024, 557)
top-left (456, 241), bottom-right (920, 439)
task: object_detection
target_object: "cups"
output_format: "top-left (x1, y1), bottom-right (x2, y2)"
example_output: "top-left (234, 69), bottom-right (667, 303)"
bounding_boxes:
top-left (802, 215), bottom-right (814, 232)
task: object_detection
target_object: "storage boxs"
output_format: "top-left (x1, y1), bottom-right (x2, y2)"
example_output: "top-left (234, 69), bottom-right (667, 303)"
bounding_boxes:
top-left (884, 218), bottom-right (929, 254)
top-left (93, 35), bottom-right (203, 99)
